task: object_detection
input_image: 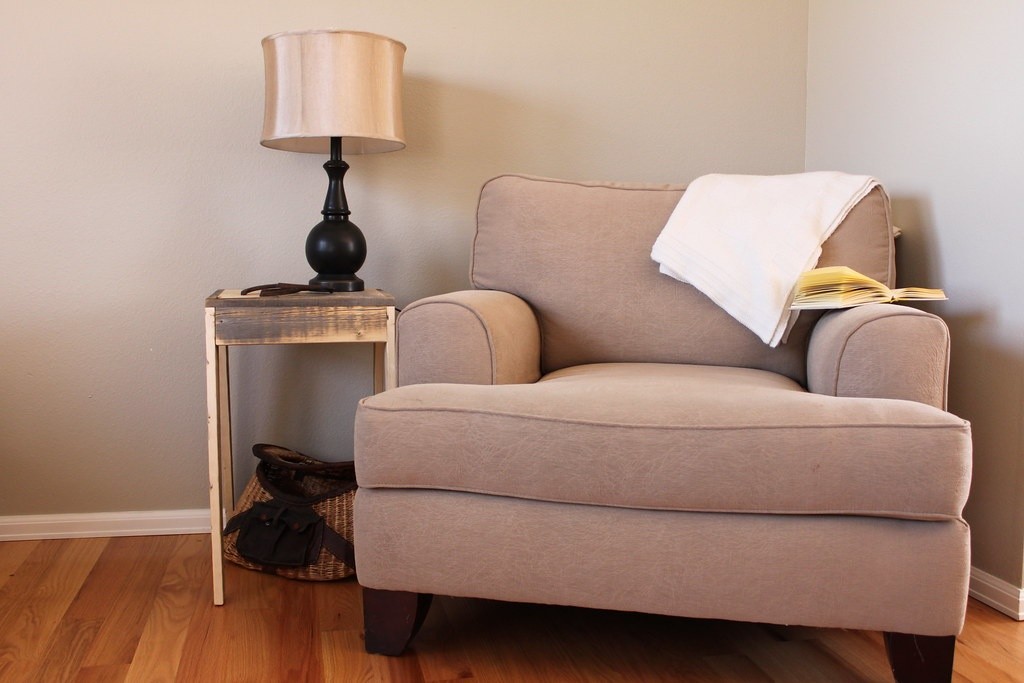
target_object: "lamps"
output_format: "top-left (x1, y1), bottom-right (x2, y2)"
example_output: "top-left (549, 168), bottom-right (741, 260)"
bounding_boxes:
top-left (260, 29), bottom-right (407, 292)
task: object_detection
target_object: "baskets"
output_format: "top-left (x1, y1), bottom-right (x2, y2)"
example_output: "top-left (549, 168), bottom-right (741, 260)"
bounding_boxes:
top-left (222, 444), bottom-right (356, 582)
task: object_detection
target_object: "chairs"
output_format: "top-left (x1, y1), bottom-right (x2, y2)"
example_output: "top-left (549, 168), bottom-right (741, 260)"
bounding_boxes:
top-left (349, 173), bottom-right (974, 683)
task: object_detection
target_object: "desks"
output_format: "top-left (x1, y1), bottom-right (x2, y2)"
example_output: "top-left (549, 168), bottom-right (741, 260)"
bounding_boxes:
top-left (203, 289), bottom-right (400, 606)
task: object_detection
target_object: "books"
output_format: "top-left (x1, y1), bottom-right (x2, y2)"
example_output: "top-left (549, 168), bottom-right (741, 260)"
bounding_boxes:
top-left (786, 266), bottom-right (951, 310)
